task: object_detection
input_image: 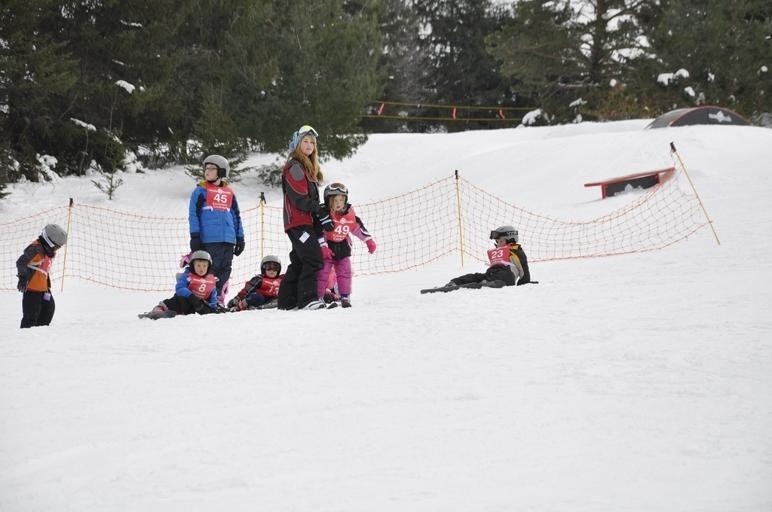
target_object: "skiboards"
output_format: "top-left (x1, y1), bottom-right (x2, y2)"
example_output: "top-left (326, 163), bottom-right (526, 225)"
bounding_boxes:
top-left (420, 280), bottom-right (504, 294)
top-left (139, 311), bottom-right (175, 319)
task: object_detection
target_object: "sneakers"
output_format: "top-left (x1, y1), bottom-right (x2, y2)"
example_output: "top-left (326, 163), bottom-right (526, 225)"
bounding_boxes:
top-left (217, 304), bottom-right (224, 310)
top-left (230, 299), bottom-right (248, 312)
top-left (286, 290), bottom-right (351, 310)
top-left (444, 281), bottom-right (456, 288)
top-left (151, 305), bottom-right (165, 313)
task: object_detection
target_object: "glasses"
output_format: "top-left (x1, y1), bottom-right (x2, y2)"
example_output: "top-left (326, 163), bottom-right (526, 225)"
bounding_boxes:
top-left (266, 266), bottom-right (279, 271)
top-left (329, 183), bottom-right (346, 192)
top-left (299, 126), bottom-right (318, 137)
top-left (491, 231), bottom-right (500, 239)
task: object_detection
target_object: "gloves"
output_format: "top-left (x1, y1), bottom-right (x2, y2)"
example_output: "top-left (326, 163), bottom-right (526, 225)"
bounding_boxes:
top-left (319, 214), bottom-right (334, 231)
top-left (188, 294), bottom-right (204, 311)
top-left (228, 296), bottom-right (241, 307)
top-left (233, 240), bottom-right (245, 256)
top-left (191, 232), bottom-right (200, 251)
top-left (320, 242), bottom-right (335, 260)
top-left (366, 239), bottom-right (376, 253)
top-left (18, 273), bottom-right (27, 292)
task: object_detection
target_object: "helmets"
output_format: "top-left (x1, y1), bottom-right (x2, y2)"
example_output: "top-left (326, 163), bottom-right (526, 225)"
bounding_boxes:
top-left (190, 250), bottom-right (213, 266)
top-left (324, 184), bottom-right (348, 207)
top-left (289, 129), bottom-right (315, 150)
top-left (261, 256), bottom-right (282, 277)
top-left (41, 224), bottom-right (67, 251)
top-left (495, 227), bottom-right (518, 244)
top-left (204, 155), bottom-right (230, 178)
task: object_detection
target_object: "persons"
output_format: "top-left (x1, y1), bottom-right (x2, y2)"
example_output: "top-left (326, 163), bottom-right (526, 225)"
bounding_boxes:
top-left (149, 249), bottom-right (219, 317)
top-left (226, 253), bottom-right (285, 313)
top-left (419, 224), bottom-right (538, 292)
top-left (188, 154), bottom-right (244, 313)
top-left (315, 182), bottom-right (376, 307)
top-left (17, 223), bottom-right (67, 329)
top-left (277, 125), bottom-right (334, 312)
